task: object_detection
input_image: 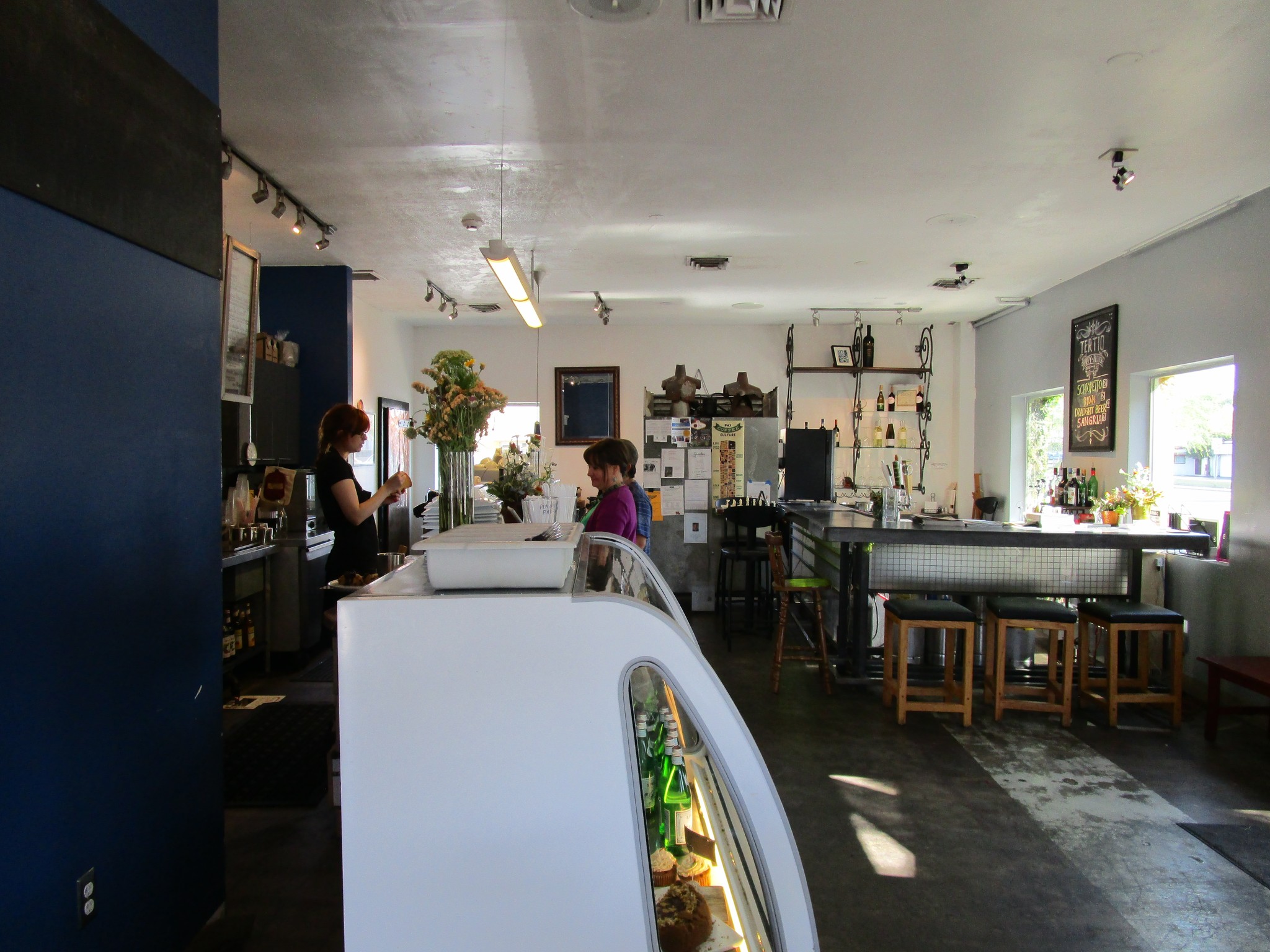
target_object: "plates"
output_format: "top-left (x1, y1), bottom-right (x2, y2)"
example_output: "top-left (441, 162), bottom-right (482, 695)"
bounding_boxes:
top-left (327, 579), bottom-right (363, 591)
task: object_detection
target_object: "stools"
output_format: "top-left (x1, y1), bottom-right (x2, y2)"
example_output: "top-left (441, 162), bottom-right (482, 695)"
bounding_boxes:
top-left (982, 593), bottom-right (1077, 729)
top-left (881, 595), bottom-right (975, 727)
top-left (1075, 599), bottom-right (1185, 728)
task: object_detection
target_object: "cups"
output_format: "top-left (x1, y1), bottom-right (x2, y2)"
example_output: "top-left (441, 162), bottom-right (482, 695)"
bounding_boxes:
top-left (376, 552), bottom-right (405, 577)
top-left (521, 496), bottom-right (577, 522)
top-left (222, 473), bottom-right (251, 524)
top-left (223, 522), bottom-right (273, 544)
top-left (941, 507), bottom-right (948, 513)
top-left (882, 488), bottom-right (901, 523)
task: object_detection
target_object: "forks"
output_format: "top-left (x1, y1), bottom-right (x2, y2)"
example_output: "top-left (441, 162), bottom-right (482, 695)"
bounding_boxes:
top-left (524, 520), bottom-right (563, 541)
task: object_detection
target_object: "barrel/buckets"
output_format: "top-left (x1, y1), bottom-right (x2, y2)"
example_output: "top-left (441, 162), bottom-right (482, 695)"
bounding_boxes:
top-left (889, 593), bottom-right (1035, 669)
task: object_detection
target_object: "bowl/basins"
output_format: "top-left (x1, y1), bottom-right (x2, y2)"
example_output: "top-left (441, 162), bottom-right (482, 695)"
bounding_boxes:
top-left (839, 501), bottom-right (873, 512)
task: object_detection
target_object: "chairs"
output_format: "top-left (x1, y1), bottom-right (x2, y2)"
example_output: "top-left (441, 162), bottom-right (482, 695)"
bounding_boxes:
top-left (714, 493), bottom-right (785, 650)
top-left (765, 525), bottom-right (836, 695)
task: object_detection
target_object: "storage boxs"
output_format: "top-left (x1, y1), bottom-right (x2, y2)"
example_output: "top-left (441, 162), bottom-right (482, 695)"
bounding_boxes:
top-left (413, 516), bottom-right (588, 594)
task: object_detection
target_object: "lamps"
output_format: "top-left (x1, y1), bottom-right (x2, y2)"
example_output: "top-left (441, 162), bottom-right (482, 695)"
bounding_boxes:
top-left (1097, 146), bottom-right (1138, 191)
top-left (811, 309), bottom-right (821, 327)
top-left (854, 310), bottom-right (862, 328)
top-left (314, 226), bottom-right (330, 251)
top-left (252, 173), bottom-right (271, 205)
top-left (949, 262), bottom-right (973, 291)
top-left (271, 189), bottom-right (288, 219)
top-left (292, 207), bottom-right (308, 234)
top-left (479, 163), bottom-right (548, 329)
top-left (438, 296), bottom-right (448, 312)
top-left (598, 307), bottom-right (607, 319)
top-left (594, 294), bottom-right (603, 312)
top-left (895, 309), bottom-right (904, 326)
top-left (424, 283), bottom-right (434, 302)
top-left (448, 303), bottom-right (459, 320)
top-left (221, 151), bottom-right (235, 181)
top-left (603, 312), bottom-right (610, 325)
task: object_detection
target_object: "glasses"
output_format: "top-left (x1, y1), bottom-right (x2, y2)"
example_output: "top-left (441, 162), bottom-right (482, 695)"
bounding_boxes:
top-left (350, 430), bottom-right (364, 438)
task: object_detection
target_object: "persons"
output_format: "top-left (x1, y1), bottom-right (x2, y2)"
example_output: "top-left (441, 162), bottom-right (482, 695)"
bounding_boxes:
top-left (579, 437), bottom-right (652, 559)
top-left (315, 404), bottom-right (406, 612)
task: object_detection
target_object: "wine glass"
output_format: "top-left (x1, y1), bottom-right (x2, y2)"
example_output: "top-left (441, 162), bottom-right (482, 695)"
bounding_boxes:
top-left (907, 428), bottom-right (918, 448)
top-left (860, 427), bottom-right (871, 447)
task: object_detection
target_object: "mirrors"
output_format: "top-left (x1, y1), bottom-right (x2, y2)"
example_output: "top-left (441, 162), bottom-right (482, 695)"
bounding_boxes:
top-left (553, 364), bottom-right (623, 448)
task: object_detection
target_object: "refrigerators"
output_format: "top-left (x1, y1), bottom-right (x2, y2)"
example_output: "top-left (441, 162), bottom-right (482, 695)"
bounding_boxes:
top-left (778, 428), bottom-right (835, 501)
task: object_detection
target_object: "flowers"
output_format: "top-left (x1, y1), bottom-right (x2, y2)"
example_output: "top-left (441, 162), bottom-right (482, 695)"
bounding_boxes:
top-left (479, 430), bottom-right (557, 505)
top-left (397, 343), bottom-right (507, 527)
top-left (1087, 458), bottom-right (1163, 516)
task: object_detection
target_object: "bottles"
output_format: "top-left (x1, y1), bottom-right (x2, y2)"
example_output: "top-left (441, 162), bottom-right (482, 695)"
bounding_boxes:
top-left (1049, 466), bottom-right (1098, 507)
top-left (805, 422), bottom-right (808, 429)
top-left (634, 704), bottom-right (693, 858)
top-left (897, 420), bottom-right (907, 448)
top-left (916, 386), bottom-right (923, 412)
top-left (885, 418), bottom-right (895, 448)
top-left (819, 419), bottom-right (826, 430)
top-left (874, 420), bottom-right (883, 448)
top-left (833, 419), bottom-right (839, 447)
top-left (888, 387), bottom-right (896, 411)
top-left (876, 385), bottom-right (885, 411)
top-left (863, 325), bottom-right (874, 367)
top-left (223, 602), bottom-right (255, 666)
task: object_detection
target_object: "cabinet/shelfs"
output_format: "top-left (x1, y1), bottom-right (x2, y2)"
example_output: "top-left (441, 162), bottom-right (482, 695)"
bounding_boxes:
top-left (785, 323), bottom-right (932, 497)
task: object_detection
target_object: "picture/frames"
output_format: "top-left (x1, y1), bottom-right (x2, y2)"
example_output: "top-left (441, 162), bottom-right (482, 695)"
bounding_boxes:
top-left (832, 342), bottom-right (857, 368)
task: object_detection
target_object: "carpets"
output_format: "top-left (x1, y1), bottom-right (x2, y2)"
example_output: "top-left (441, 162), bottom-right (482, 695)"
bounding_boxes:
top-left (1178, 816), bottom-right (1270, 890)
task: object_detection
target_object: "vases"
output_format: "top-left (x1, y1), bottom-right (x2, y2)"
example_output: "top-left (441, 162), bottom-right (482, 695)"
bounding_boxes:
top-left (1129, 501), bottom-right (1151, 523)
top-left (1101, 509), bottom-right (1118, 526)
top-left (436, 441), bottom-right (479, 533)
top-left (505, 501), bottom-right (523, 521)
